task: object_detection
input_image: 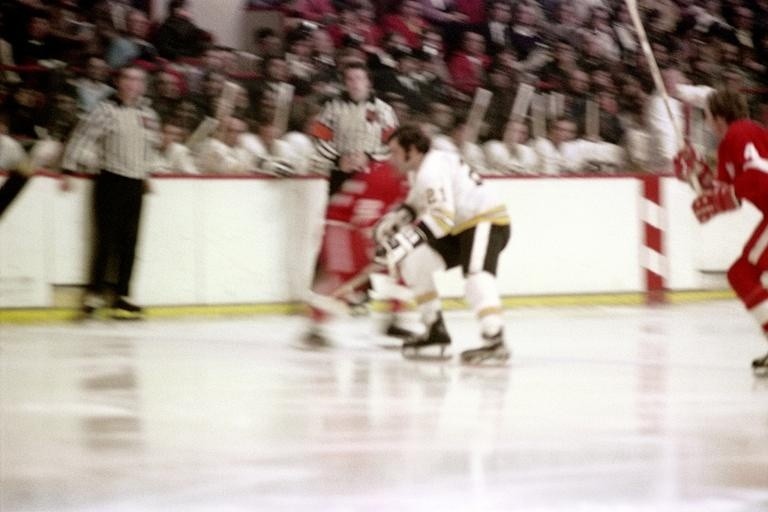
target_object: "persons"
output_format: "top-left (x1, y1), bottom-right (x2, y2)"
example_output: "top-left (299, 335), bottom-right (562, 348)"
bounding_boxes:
top-left (669, 88), bottom-right (768, 374)
top-left (302, 54), bottom-right (398, 321)
top-left (294, 126), bottom-right (411, 351)
top-left (1, 2), bottom-right (767, 186)
top-left (378, 125), bottom-right (514, 364)
top-left (56, 59), bottom-right (159, 316)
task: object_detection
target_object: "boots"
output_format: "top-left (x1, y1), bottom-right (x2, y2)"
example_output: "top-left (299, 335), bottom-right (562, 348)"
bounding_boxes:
top-left (460, 328), bottom-right (505, 360)
top-left (401, 310), bottom-right (450, 348)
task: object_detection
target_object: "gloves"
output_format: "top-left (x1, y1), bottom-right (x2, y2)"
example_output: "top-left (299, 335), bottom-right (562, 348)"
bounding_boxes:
top-left (690, 184), bottom-right (742, 225)
top-left (256, 156), bottom-right (294, 180)
top-left (673, 138), bottom-right (711, 186)
top-left (371, 203), bottom-right (416, 245)
top-left (373, 223), bottom-right (426, 267)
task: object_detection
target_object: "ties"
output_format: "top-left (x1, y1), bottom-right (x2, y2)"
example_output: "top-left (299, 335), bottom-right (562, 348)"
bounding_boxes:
top-left (682, 102), bottom-right (691, 139)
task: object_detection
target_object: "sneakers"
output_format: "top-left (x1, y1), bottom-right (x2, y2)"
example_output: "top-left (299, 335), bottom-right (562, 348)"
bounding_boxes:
top-left (83, 290), bottom-right (103, 314)
top-left (109, 297), bottom-right (141, 320)
top-left (749, 354), bottom-right (767, 377)
top-left (367, 325), bottom-right (414, 346)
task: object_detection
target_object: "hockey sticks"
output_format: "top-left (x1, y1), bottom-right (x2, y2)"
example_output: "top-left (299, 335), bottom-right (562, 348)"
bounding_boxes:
top-left (297, 262), bottom-right (387, 316)
